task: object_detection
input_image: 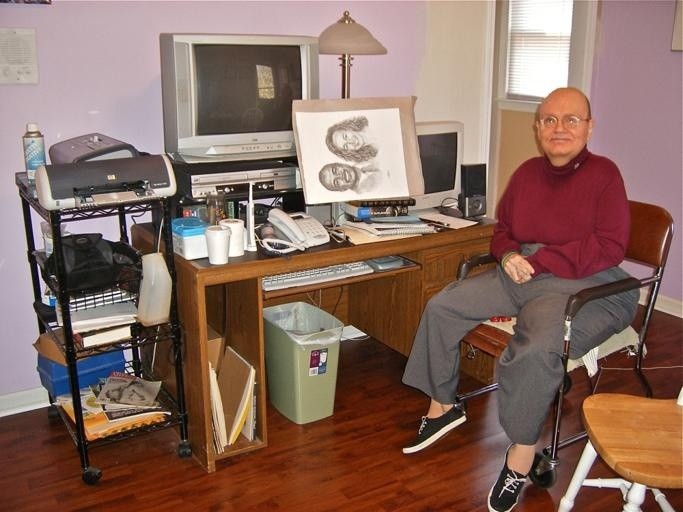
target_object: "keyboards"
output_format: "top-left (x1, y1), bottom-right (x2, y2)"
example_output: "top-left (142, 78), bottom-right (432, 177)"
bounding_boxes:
top-left (262, 261), bottom-right (374, 292)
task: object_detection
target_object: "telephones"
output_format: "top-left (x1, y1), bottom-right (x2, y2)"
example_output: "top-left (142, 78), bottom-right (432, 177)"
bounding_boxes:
top-left (267, 208), bottom-right (330, 249)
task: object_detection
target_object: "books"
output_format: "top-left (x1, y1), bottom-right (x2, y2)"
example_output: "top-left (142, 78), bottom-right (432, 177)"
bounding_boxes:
top-left (206, 347), bottom-right (258, 455)
top-left (335, 195), bottom-right (436, 245)
top-left (50, 287), bottom-right (138, 351)
top-left (88, 370), bottom-right (172, 423)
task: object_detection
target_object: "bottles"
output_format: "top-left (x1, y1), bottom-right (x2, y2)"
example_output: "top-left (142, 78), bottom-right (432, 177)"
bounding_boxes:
top-left (21, 122), bottom-right (46, 202)
top-left (207, 195), bottom-right (226, 225)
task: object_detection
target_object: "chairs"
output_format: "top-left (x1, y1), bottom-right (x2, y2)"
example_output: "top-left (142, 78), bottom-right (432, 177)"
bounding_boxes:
top-left (458, 197), bottom-right (674, 488)
top-left (550, 390), bottom-right (681, 511)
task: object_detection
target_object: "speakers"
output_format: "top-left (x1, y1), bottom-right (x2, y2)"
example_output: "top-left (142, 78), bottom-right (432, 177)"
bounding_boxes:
top-left (460, 163), bottom-right (487, 217)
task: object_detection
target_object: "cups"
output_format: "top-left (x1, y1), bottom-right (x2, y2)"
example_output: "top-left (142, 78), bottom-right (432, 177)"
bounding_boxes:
top-left (204, 226), bottom-right (232, 267)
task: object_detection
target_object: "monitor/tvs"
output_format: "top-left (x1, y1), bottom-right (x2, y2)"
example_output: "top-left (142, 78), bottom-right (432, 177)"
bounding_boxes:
top-left (407, 122), bottom-right (464, 216)
top-left (160, 32), bottom-right (320, 165)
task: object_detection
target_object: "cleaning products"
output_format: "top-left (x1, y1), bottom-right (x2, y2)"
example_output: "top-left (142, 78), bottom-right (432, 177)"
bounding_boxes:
top-left (21, 122), bottom-right (47, 188)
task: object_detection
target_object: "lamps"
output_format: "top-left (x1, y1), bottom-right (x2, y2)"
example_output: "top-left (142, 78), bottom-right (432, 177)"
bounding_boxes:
top-left (318, 9), bottom-right (388, 99)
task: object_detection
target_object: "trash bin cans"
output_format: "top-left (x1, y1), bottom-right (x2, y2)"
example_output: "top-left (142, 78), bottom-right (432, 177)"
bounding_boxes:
top-left (263, 301), bottom-right (344, 425)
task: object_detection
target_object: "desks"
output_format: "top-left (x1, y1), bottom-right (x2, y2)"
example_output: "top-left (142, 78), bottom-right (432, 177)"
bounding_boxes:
top-left (131, 201), bottom-right (501, 473)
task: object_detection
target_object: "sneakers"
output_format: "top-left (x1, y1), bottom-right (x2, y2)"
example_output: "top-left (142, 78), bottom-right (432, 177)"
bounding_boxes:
top-left (402, 404), bottom-right (466, 454)
top-left (487, 442), bottom-right (530, 512)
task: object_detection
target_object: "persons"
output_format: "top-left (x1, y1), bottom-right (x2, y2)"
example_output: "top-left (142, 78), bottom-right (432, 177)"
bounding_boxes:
top-left (325, 114), bottom-right (380, 162)
top-left (318, 161), bottom-right (392, 196)
top-left (401, 86), bottom-right (633, 512)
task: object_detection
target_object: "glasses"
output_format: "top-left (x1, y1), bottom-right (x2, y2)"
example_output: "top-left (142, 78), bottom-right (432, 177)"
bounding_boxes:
top-left (537, 115), bottom-right (589, 130)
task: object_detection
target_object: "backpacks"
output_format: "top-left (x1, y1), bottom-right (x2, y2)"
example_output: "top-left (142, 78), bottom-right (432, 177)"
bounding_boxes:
top-left (46, 234), bottom-right (141, 293)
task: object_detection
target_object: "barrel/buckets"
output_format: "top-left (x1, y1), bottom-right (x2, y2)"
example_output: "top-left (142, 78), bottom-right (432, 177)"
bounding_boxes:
top-left (133, 253), bottom-right (173, 326)
